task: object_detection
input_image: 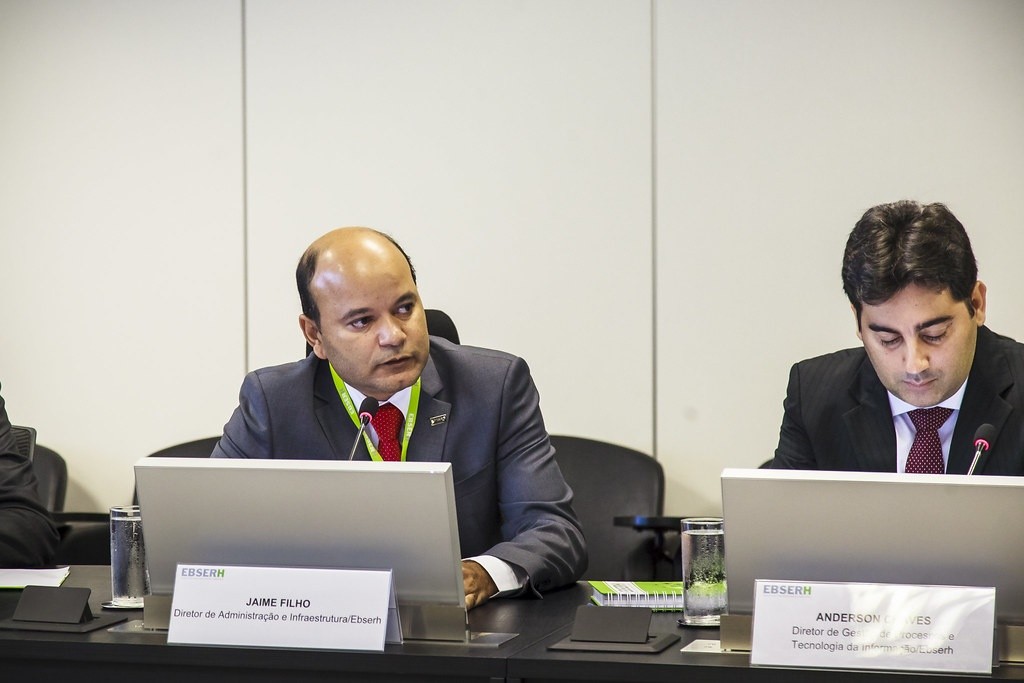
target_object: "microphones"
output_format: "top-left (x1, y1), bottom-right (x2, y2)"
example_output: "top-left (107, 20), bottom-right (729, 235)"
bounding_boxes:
top-left (967, 422), bottom-right (996, 476)
top-left (349, 396), bottom-right (378, 461)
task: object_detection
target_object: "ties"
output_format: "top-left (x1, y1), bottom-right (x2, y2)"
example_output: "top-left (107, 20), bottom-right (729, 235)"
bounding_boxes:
top-left (370, 402), bottom-right (404, 461)
top-left (904, 407), bottom-right (954, 474)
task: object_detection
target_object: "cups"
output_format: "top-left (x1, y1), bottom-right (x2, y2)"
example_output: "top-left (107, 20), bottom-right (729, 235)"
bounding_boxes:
top-left (680, 517), bottom-right (729, 624)
top-left (110, 505), bottom-right (151, 606)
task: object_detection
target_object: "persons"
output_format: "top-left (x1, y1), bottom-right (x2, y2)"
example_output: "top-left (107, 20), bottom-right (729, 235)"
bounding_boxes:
top-left (209, 227), bottom-right (587, 609)
top-left (759, 200), bottom-right (1024, 478)
top-left (0, 382), bottom-right (60, 569)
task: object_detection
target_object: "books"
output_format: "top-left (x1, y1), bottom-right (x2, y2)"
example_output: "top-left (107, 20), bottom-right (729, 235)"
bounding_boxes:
top-left (589, 581), bottom-right (727, 611)
